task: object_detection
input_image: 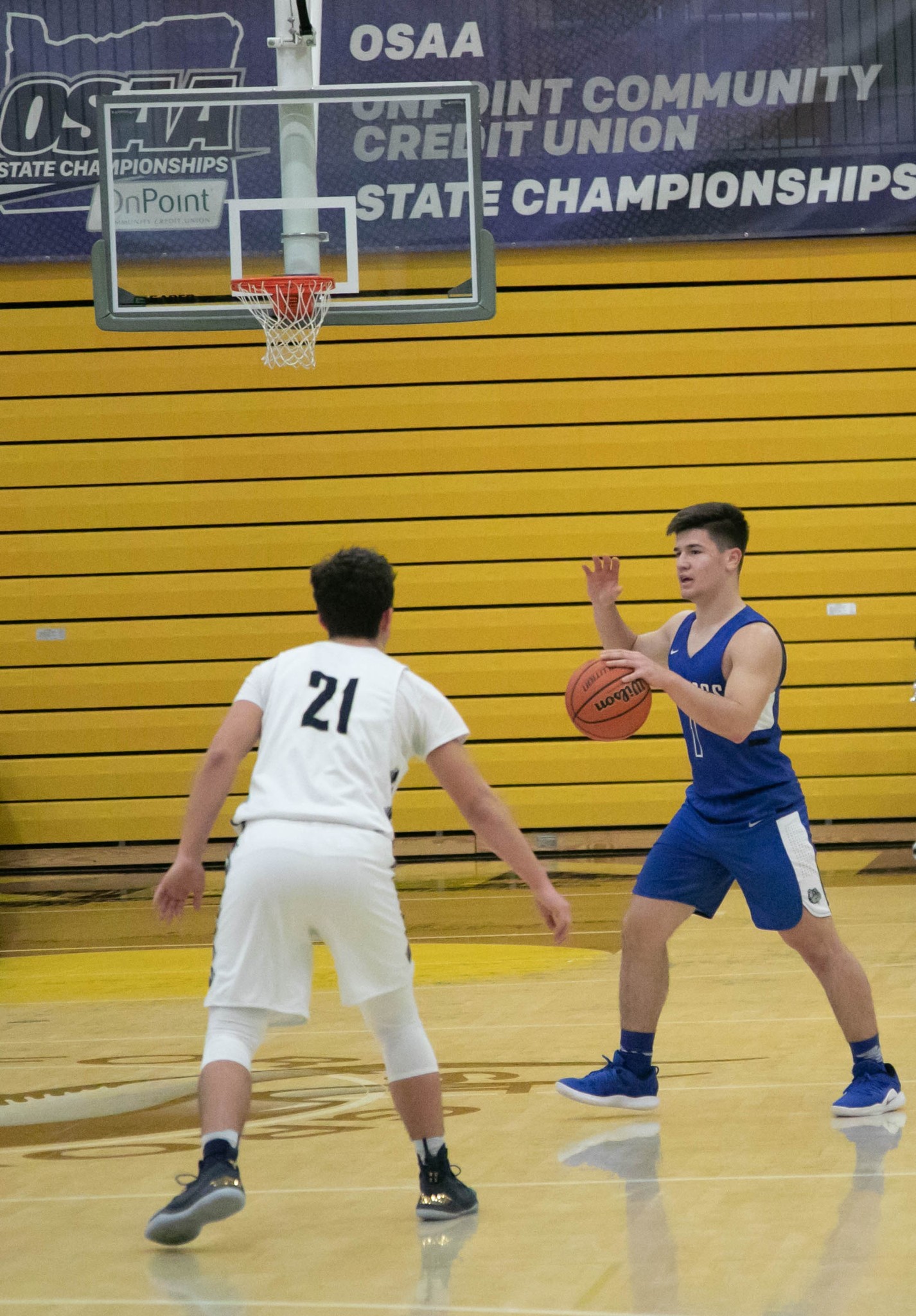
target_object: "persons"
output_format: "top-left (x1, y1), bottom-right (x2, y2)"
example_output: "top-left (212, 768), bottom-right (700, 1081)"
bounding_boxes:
top-left (556, 501), bottom-right (906, 1117)
top-left (144, 544), bottom-right (570, 1245)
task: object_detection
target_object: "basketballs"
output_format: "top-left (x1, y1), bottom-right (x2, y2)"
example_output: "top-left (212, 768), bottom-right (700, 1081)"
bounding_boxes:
top-left (563, 656), bottom-right (652, 743)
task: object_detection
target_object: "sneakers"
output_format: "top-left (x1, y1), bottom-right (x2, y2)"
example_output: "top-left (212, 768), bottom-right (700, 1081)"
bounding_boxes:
top-left (556, 1050), bottom-right (660, 1110)
top-left (414, 1137), bottom-right (479, 1219)
top-left (142, 1157), bottom-right (247, 1244)
top-left (829, 1060), bottom-right (905, 1117)
